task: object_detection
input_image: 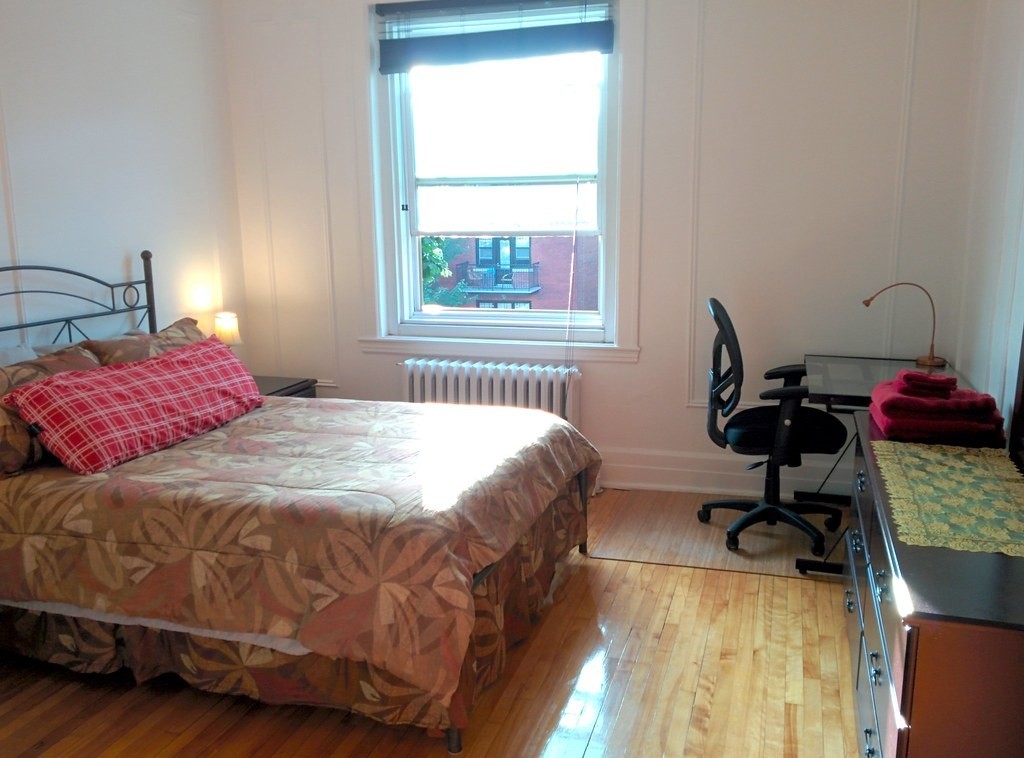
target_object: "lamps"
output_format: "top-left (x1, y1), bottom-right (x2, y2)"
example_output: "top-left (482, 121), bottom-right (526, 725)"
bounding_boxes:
top-left (863, 282), bottom-right (947, 367)
top-left (214, 312), bottom-right (243, 355)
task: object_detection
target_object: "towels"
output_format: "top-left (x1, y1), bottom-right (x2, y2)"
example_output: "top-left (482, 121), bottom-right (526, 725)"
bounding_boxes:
top-left (869, 368), bottom-right (1004, 440)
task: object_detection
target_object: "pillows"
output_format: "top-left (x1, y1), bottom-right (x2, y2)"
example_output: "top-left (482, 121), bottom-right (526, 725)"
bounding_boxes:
top-left (0, 333), bottom-right (267, 477)
top-left (0, 318), bottom-right (204, 480)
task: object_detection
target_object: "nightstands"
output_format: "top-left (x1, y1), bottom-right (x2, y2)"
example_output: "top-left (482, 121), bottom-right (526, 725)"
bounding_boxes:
top-left (252, 375), bottom-right (318, 399)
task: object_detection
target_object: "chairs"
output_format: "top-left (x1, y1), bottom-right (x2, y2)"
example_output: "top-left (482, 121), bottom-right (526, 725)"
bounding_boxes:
top-left (501, 273), bottom-right (513, 288)
top-left (468, 270), bottom-right (483, 288)
top-left (698, 297), bottom-right (848, 557)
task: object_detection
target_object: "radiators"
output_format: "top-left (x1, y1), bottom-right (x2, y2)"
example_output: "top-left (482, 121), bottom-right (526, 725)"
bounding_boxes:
top-left (397, 357), bottom-right (582, 432)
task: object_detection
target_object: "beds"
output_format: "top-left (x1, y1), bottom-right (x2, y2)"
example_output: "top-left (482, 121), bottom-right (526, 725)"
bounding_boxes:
top-left (0, 250), bottom-right (601, 758)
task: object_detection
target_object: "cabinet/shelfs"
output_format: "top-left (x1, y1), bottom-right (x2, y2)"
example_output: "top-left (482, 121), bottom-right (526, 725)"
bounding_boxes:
top-left (839, 409), bottom-right (1024, 758)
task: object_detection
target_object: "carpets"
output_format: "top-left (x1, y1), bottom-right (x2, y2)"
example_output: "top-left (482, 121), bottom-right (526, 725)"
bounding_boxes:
top-left (590, 489), bottom-right (833, 580)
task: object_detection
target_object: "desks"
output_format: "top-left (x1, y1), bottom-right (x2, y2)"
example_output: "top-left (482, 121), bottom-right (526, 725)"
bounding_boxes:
top-left (793, 355), bottom-right (979, 575)
top-left (470, 268), bottom-right (495, 289)
